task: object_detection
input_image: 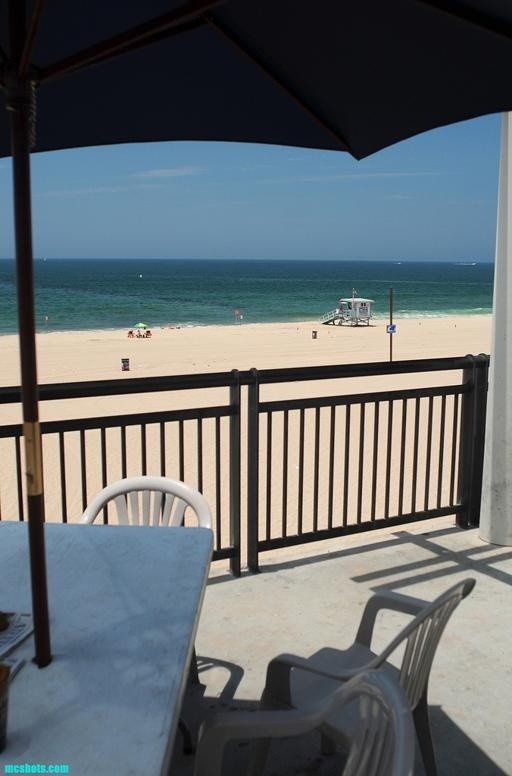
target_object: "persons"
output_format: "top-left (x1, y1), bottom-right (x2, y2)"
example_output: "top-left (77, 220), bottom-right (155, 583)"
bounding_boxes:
top-left (136, 330), bottom-right (142, 335)
top-left (142, 329), bottom-right (151, 338)
top-left (128, 328), bottom-right (134, 338)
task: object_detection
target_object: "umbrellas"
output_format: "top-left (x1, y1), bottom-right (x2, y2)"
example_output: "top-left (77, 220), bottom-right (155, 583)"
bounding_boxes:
top-left (132, 321), bottom-right (150, 328)
top-left (1, 3), bottom-right (512, 670)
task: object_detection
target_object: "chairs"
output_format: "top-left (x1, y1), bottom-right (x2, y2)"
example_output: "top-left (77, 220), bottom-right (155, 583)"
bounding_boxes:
top-left (76, 476), bottom-right (212, 527)
top-left (188, 666), bottom-right (424, 775)
top-left (270, 572), bottom-right (476, 774)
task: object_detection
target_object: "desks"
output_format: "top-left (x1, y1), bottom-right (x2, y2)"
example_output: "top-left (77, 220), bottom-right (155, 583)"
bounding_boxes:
top-left (0, 520), bottom-right (213, 775)
